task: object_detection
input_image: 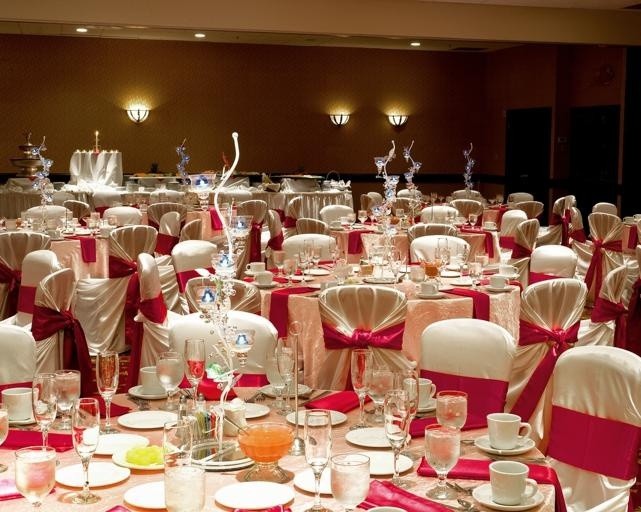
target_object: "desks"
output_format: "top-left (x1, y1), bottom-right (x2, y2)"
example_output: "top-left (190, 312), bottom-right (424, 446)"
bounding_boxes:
top-left (68, 150), bottom-right (124, 187)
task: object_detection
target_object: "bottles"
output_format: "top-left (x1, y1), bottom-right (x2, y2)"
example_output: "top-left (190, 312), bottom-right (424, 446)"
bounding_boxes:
top-left (322, 180), bottom-right (331, 191)
top-left (104, 219), bottom-right (108, 225)
top-left (99, 221), bottom-right (104, 228)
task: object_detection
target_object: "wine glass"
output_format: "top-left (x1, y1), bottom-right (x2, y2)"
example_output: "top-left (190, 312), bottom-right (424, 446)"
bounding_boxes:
top-left (468, 260), bottom-right (482, 292)
top-left (283, 258), bottom-right (299, 287)
top-left (0, 402), bottom-right (11, 473)
top-left (455, 247), bottom-right (468, 280)
top-left (31, 371), bottom-right (60, 454)
top-left (301, 408), bottom-right (332, 511)
top-left (488, 196), bottom-right (496, 206)
top-left (14, 443), bottom-right (57, 512)
top-left (438, 237), bottom-right (449, 264)
top-left (396, 209), bottom-right (405, 231)
top-left (162, 421), bottom-right (193, 465)
top-left (395, 370), bottom-right (424, 460)
top-left (351, 348), bottom-right (374, 429)
top-left (384, 388), bottom-right (406, 489)
top-left (448, 210), bottom-right (457, 226)
top-left (276, 337), bottom-right (301, 416)
top-left (331, 451), bottom-right (371, 512)
top-left (266, 357), bottom-right (292, 413)
top-left (389, 261), bottom-right (402, 290)
top-left (72, 216), bottom-right (79, 230)
top-left (57, 368), bottom-right (80, 431)
top-left (87, 218), bottom-right (97, 238)
top-left (347, 212), bottom-right (356, 230)
top-left (328, 243), bottom-right (341, 267)
top-left (357, 210), bottom-right (368, 229)
top-left (362, 367), bottom-right (393, 423)
top-left (438, 195), bottom-right (446, 205)
top-left (96, 351), bottom-right (120, 434)
top-left (184, 338), bottom-right (205, 412)
top-left (469, 213), bottom-right (478, 229)
top-left (60, 214), bottom-right (66, 231)
top-left (156, 352), bottom-right (183, 410)
top-left (425, 425), bottom-right (461, 499)
top-left (21, 211), bottom-right (29, 230)
top-left (313, 245), bottom-right (322, 267)
top-left (272, 250), bottom-right (287, 277)
top-left (497, 196), bottom-right (503, 208)
top-left (330, 264), bottom-right (353, 286)
top-left (297, 250), bottom-right (307, 281)
top-left (420, 257), bottom-right (442, 283)
top-left (72, 397), bottom-right (102, 505)
top-left (303, 239), bottom-right (313, 267)
top-left (237, 422), bottom-right (297, 483)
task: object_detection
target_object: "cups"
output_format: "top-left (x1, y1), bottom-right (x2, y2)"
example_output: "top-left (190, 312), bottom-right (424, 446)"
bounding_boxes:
top-left (420, 281), bottom-right (437, 294)
top-left (330, 221), bottom-right (341, 227)
top-left (221, 202), bottom-right (230, 209)
top-left (108, 215), bottom-right (117, 226)
top-left (246, 262), bottom-right (266, 273)
top-left (1, 387), bottom-right (33, 421)
top-left (475, 251), bottom-right (489, 266)
top-left (199, 200), bottom-right (210, 211)
top-left (140, 366), bottom-right (163, 396)
top-left (410, 266), bottom-right (426, 282)
top-left (45, 215), bottom-right (57, 233)
top-left (166, 467), bottom-right (208, 511)
top-left (487, 461), bottom-right (539, 504)
top-left (487, 412), bottom-right (532, 450)
top-left (485, 220), bottom-right (497, 230)
top-left (91, 213), bottom-right (101, 222)
top-left (404, 377), bottom-right (437, 408)
top-left (500, 264), bottom-right (518, 279)
top-left (140, 204), bottom-right (147, 213)
top-left (254, 271), bottom-right (271, 285)
top-left (437, 389), bottom-right (468, 432)
top-left (5, 220), bottom-right (17, 230)
top-left (338, 215), bottom-right (348, 225)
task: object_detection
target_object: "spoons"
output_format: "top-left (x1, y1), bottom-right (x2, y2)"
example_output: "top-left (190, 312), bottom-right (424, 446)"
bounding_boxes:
top-left (462, 439), bottom-right (474, 444)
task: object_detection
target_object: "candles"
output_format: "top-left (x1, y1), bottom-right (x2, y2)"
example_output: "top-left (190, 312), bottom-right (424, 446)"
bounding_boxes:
top-left (94, 130), bottom-right (99, 149)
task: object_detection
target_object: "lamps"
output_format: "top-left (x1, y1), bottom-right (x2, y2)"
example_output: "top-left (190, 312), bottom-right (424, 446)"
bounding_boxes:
top-left (388, 114), bottom-right (410, 127)
top-left (124, 108), bottom-right (153, 124)
top-left (328, 113), bottom-right (351, 127)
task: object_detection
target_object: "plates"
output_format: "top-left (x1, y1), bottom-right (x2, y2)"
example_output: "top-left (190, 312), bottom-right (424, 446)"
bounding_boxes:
top-left (293, 464), bottom-right (330, 496)
top-left (111, 448), bottom-right (167, 471)
top-left (360, 450), bottom-right (413, 475)
top-left (259, 383), bottom-right (310, 400)
top-left (344, 427), bottom-right (412, 448)
top-left (56, 459), bottom-right (132, 488)
top-left (125, 481), bottom-right (166, 510)
top-left (214, 481), bottom-right (296, 509)
top-left (418, 396), bottom-right (441, 412)
top-left (118, 411), bottom-right (181, 429)
top-left (350, 263), bottom-right (359, 272)
top-left (215, 401), bottom-right (269, 420)
top-left (473, 435), bottom-right (535, 456)
top-left (286, 407), bottom-right (348, 427)
top-left (127, 385), bottom-right (179, 398)
top-left (483, 227), bottom-right (498, 231)
top-left (493, 273), bottom-right (521, 280)
top-left (331, 226), bottom-right (342, 230)
top-left (3, 416), bottom-right (39, 424)
top-left (451, 278), bottom-right (472, 286)
top-left (74, 229), bottom-right (90, 236)
top-left (471, 482), bottom-right (546, 511)
top-left (304, 283), bottom-right (321, 289)
top-left (373, 229), bottom-right (382, 234)
top-left (301, 267), bottom-right (328, 276)
top-left (441, 271), bottom-right (459, 277)
top-left (50, 237), bottom-right (64, 241)
top-left (417, 292), bottom-right (443, 299)
top-left (63, 231), bottom-right (73, 235)
top-left (95, 433), bottom-right (149, 455)
top-left (438, 284), bottom-right (453, 291)
top-left (251, 281), bottom-right (278, 289)
top-left (363, 277), bottom-right (398, 285)
top-left (485, 284), bottom-right (514, 292)
top-left (447, 264), bottom-right (468, 271)
top-left (244, 270), bottom-right (271, 276)
